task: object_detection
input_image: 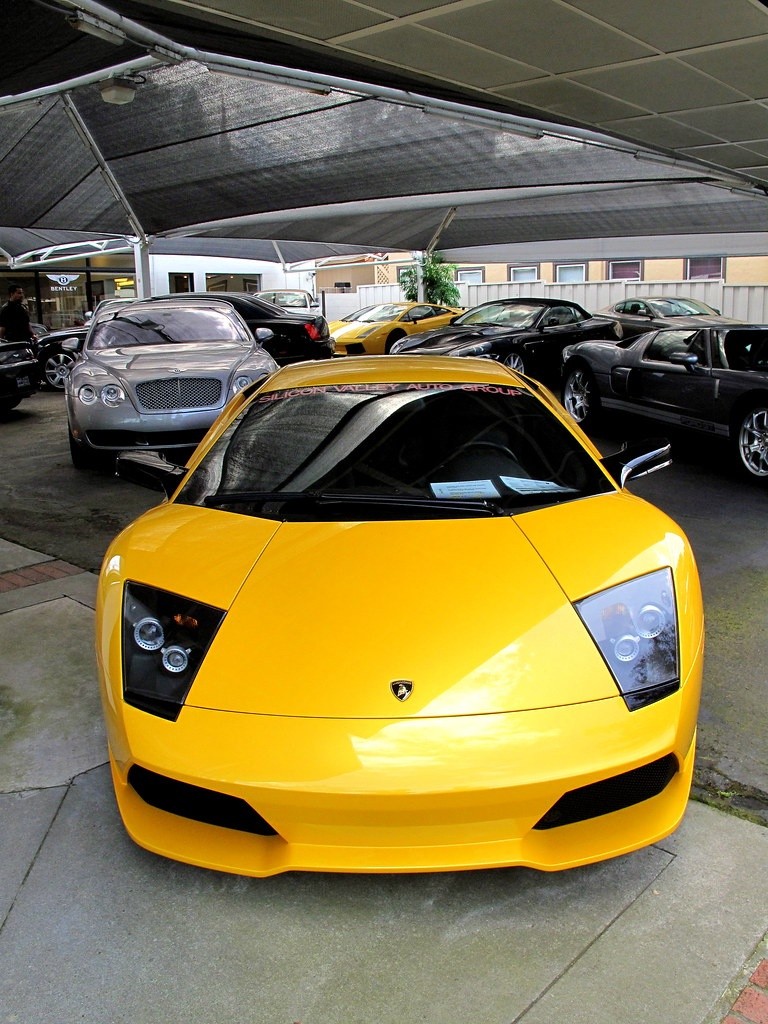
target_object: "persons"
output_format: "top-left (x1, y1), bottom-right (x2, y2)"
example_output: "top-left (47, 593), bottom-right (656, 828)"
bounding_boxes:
top-left (0, 284), bottom-right (39, 346)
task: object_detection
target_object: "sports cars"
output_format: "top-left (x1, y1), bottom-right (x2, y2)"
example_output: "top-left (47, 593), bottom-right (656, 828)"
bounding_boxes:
top-left (327, 302), bottom-right (476, 357)
top-left (93, 355), bottom-right (705, 880)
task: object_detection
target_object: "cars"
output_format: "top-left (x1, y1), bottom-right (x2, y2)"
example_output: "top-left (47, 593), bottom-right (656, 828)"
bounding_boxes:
top-left (82, 297), bottom-right (138, 328)
top-left (558, 324), bottom-right (768, 482)
top-left (589, 295), bottom-right (751, 337)
top-left (33, 291), bottom-right (338, 391)
top-left (0, 337), bottom-right (38, 416)
top-left (63, 298), bottom-right (282, 470)
top-left (252, 288), bottom-right (322, 315)
top-left (389, 298), bottom-right (624, 375)
